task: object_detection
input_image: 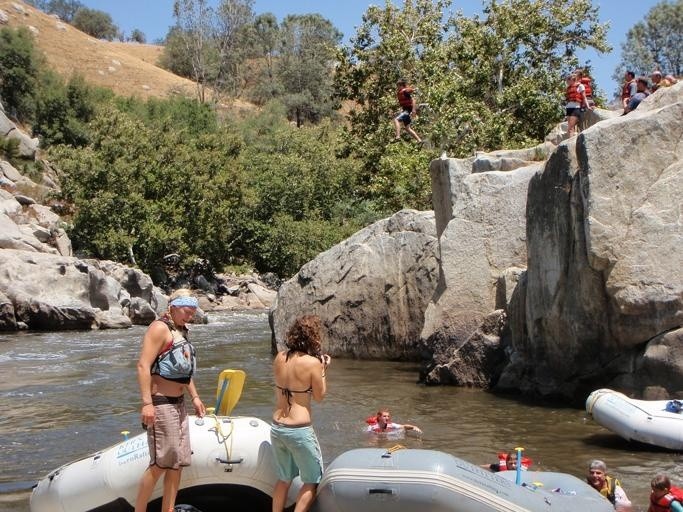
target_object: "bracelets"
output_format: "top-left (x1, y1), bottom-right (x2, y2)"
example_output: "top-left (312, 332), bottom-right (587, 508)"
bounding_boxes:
top-left (190, 395), bottom-right (198, 401)
top-left (140, 399), bottom-right (153, 408)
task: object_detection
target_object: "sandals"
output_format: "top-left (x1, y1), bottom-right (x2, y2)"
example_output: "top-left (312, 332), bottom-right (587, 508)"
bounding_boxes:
top-left (390, 137), bottom-right (401, 144)
top-left (417, 140), bottom-right (424, 151)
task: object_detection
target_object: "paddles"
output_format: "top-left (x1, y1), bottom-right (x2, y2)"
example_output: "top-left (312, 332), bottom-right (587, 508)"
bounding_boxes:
top-left (217, 369), bottom-right (246, 416)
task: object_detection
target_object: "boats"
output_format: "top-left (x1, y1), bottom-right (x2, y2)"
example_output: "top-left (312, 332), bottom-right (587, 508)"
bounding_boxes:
top-left (314, 445), bottom-right (618, 511)
top-left (26, 412), bottom-right (323, 512)
top-left (585, 388), bottom-right (683, 452)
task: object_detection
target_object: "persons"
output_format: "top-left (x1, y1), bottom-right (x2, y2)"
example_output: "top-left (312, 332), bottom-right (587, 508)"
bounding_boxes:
top-left (477, 450), bottom-right (532, 472)
top-left (268, 314), bottom-right (333, 512)
top-left (582, 458), bottom-right (632, 511)
top-left (132, 287), bottom-right (206, 512)
top-left (561, 66), bottom-right (679, 139)
top-left (387, 78), bottom-right (423, 146)
top-left (645, 474), bottom-right (683, 512)
top-left (363, 409), bottom-right (423, 435)
